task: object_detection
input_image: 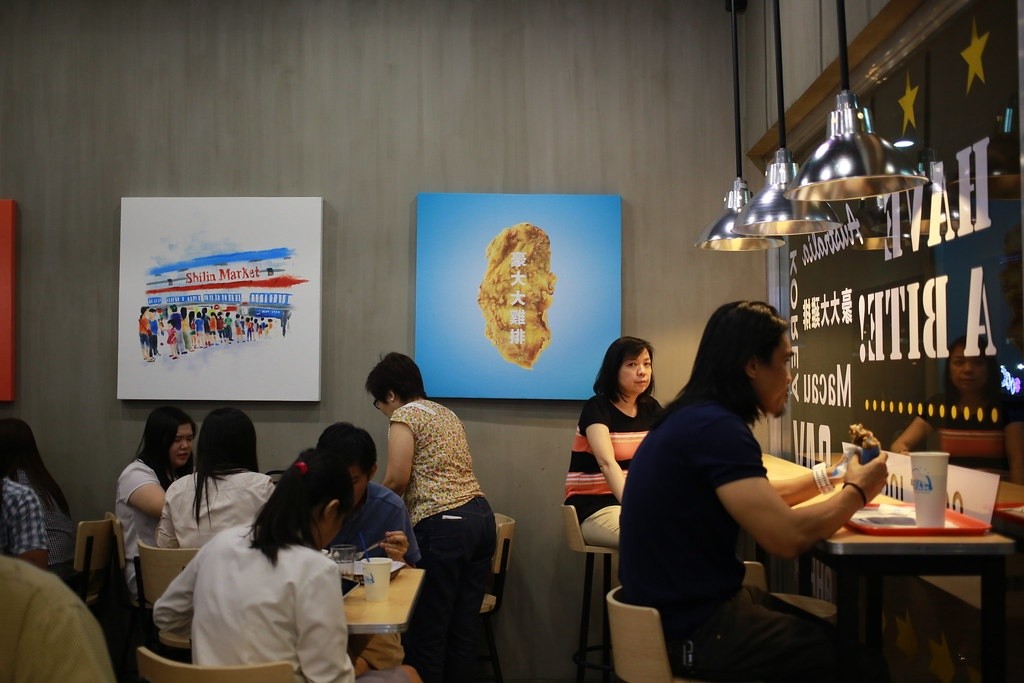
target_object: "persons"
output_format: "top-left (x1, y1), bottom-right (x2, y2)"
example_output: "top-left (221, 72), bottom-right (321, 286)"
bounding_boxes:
top-left (115, 405), bottom-right (196, 598)
top-left (317, 422), bottom-right (417, 674)
top-left (891, 332), bottom-right (1024, 494)
top-left (0, 418), bottom-right (77, 569)
top-left (562, 336), bottom-right (663, 549)
top-left (156, 406), bottom-right (276, 549)
top-left (365, 351), bottom-right (499, 670)
top-left (0, 553), bottom-right (118, 683)
top-left (0, 475), bottom-right (50, 570)
top-left (151, 449), bottom-right (418, 683)
top-left (615, 300), bottom-right (892, 683)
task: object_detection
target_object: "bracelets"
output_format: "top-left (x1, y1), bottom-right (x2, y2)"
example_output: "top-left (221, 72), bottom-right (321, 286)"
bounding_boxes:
top-left (812, 462), bottom-right (835, 495)
top-left (841, 482), bottom-right (867, 509)
top-left (896, 449), bottom-right (909, 454)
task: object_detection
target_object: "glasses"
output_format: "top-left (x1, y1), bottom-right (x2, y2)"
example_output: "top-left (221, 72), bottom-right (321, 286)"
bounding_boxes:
top-left (373, 399), bottom-right (381, 410)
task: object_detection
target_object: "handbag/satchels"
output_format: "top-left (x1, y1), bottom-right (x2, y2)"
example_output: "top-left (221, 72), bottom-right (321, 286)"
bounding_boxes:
top-left (88, 536), bottom-right (151, 682)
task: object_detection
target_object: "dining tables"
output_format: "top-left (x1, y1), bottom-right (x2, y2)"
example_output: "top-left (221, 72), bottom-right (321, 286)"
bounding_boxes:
top-left (799, 450), bottom-right (1024, 531)
top-left (343, 568), bottom-right (428, 636)
top-left (762, 454), bottom-right (1016, 683)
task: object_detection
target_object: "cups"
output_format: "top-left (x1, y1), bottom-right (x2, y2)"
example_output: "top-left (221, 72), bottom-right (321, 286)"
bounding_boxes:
top-left (362, 557), bottom-right (392, 601)
top-left (329, 544), bottom-right (356, 583)
top-left (910, 452), bottom-right (950, 528)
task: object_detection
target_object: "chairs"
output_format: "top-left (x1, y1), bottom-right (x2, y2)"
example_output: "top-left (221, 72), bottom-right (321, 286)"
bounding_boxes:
top-left (73, 505), bottom-right (838, 683)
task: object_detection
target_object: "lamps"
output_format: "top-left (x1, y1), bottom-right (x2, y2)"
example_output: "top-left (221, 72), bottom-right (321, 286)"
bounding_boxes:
top-left (697, 0), bottom-right (786, 252)
top-left (731, 0), bottom-right (843, 236)
top-left (783, 0), bottom-right (929, 202)
